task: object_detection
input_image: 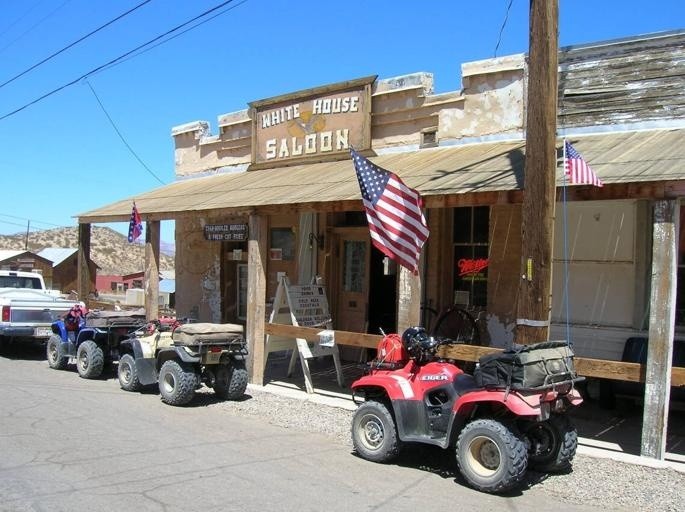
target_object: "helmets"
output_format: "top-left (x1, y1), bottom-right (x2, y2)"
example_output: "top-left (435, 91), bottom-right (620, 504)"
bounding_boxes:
top-left (401, 326), bottom-right (426, 348)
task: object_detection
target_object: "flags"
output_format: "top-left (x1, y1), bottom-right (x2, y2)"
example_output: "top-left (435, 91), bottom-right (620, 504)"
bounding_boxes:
top-left (128, 205), bottom-right (143, 243)
top-left (349, 147), bottom-right (431, 276)
top-left (565, 141), bottom-right (604, 188)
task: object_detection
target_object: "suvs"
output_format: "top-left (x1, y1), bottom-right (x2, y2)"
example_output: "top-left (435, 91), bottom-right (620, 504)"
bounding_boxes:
top-left (47, 304), bottom-right (249, 406)
top-left (350, 326), bottom-right (586, 493)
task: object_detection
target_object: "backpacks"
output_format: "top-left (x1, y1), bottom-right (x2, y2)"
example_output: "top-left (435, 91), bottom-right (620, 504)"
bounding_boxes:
top-left (474, 341), bottom-right (575, 388)
top-left (62, 303), bottom-right (87, 331)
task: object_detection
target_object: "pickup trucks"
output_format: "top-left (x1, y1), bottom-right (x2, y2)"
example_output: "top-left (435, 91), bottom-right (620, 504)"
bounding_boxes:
top-left (0, 270), bottom-right (86, 352)
